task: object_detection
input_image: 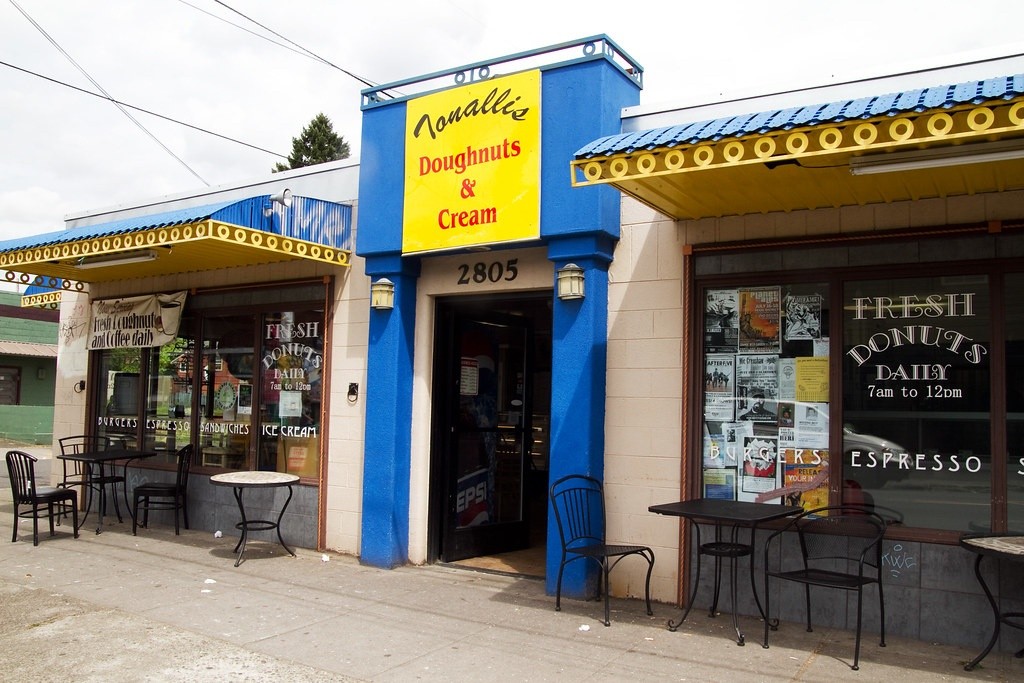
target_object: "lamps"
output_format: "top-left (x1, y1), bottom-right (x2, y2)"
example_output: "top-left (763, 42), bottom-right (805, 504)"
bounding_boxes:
top-left (555, 262), bottom-right (584, 301)
top-left (371, 278), bottom-right (394, 310)
top-left (269, 187), bottom-right (293, 208)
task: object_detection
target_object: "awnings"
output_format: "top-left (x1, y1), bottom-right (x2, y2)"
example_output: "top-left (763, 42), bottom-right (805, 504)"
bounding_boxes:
top-left (0, 194), bottom-right (351, 281)
top-left (569, 74), bottom-right (1024, 220)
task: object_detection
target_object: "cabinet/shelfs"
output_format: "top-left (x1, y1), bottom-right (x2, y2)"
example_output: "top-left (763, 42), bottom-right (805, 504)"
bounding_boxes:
top-left (491, 413), bottom-right (549, 470)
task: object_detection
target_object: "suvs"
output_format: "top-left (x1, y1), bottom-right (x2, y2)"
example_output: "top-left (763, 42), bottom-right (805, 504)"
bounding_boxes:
top-left (712, 397), bottom-right (914, 488)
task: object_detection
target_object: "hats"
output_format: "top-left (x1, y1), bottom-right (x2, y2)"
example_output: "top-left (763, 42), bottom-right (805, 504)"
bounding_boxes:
top-left (752, 388), bottom-right (764, 397)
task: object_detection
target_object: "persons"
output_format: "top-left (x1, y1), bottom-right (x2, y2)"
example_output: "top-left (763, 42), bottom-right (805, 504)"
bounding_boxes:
top-left (806, 408), bottom-right (817, 420)
top-left (739, 388), bottom-right (777, 421)
top-left (781, 409), bottom-right (792, 425)
top-left (711, 367), bottom-right (720, 387)
top-left (727, 429), bottom-right (735, 442)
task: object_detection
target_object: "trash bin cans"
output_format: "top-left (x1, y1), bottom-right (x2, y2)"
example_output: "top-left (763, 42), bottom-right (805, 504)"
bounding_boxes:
top-left (168, 404), bottom-right (184, 418)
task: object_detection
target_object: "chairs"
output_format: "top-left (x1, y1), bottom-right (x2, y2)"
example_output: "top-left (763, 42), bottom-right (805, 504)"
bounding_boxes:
top-left (131, 443), bottom-right (194, 537)
top-left (6, 450), bottom-right (79, 546)
top-left (548, 474), bottom-right (656, 627)
top-left (58, 434), bottom-right (123, 530)
top-left (761, 505), bottom-right (889, 668)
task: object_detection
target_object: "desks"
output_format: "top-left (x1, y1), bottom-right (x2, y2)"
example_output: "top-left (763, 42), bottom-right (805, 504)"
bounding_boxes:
top-left (209, 470), bottom-right (300, 567)
top-left (644, 497), bottom-right (806, 647)
top-left (956, 532), bottom-right (1024, 671)
top-left (56, 448), bottom-right (157, 536)
